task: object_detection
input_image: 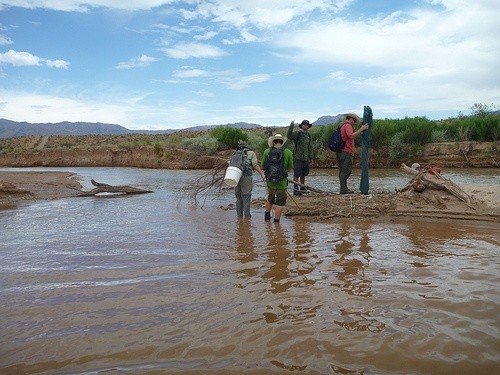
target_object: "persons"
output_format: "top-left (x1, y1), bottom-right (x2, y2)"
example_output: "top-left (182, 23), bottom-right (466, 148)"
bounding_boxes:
top-left (260, 133), bottom-right (293, 223)
top-left (336, 113), bottom-right (369, 193)
top-left (286, 120), bottom-right (313, 195)
top-left (230, 141), bottom-right (264, 219)
top-left (411, 161), bottom-right (425, 171)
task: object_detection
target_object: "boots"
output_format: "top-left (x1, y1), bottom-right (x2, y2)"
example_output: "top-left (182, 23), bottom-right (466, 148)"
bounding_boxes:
top-left (274, 218), bottom-right (279, 222)
top-left (339, 178), bottom-right (354, 194)
top-left (265, 211), bottom-right (273, 220)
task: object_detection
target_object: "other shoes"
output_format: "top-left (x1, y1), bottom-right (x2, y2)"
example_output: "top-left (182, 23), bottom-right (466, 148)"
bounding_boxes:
top-left (294, 186), bottom-right (310, 195)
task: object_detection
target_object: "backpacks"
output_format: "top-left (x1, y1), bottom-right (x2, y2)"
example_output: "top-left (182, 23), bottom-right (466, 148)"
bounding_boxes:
top-left (264, 146), bottom-right (288, 183)
top-left (328, 122), bottom-right (353, 152)
top-left (229, 149), bottom-right (255, 176)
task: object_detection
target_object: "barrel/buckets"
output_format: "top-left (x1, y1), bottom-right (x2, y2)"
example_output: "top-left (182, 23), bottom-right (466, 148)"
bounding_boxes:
top-left (224, 167), bottom-right (243, 188)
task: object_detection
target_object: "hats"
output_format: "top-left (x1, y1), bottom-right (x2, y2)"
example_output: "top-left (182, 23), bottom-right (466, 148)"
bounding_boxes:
top-left (268, 134), bottom-right (288, 147)
top-left (237, 142), bottom-right (249, 148)
top-left (299, 120), bottom-right (312, 129)
top-left (342, 112), bottom-right (360, 123)
